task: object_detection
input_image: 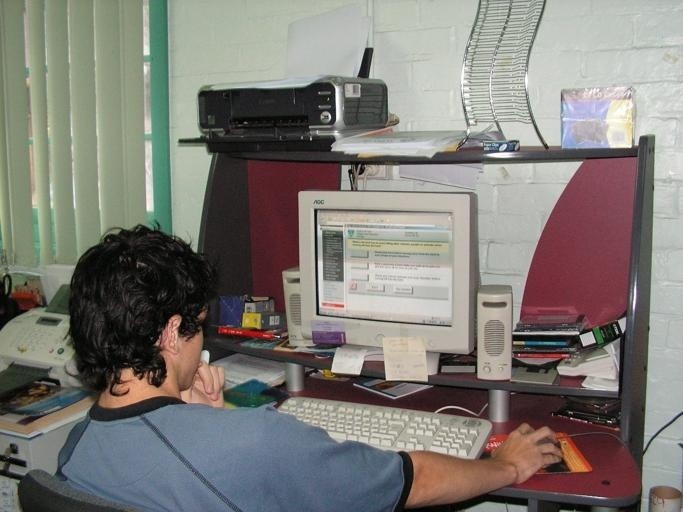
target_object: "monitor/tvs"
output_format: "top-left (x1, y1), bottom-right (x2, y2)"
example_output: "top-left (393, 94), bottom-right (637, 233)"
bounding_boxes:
top-left (298, 189), bottom-right (479, 377)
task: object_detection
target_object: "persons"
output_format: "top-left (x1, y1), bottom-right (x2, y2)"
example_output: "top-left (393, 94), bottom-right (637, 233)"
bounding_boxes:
top-left (54, 219), bottom-right (564, 512)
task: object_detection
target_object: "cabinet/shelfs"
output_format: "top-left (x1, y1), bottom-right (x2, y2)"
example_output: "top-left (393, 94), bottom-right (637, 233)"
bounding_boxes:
top-left (178, 129), bottom-right (656, 446)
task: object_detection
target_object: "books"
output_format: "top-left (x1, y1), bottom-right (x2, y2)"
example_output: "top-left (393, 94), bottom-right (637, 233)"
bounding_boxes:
top-left (511, 314), bottom-right (585, 359)
top-left (352, 378), bottom-right (434, 400)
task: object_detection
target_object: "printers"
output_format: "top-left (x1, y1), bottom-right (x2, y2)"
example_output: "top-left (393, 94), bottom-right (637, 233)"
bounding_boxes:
top-left (0, 283), bottom-right (84, 400)
top-left (176, 47), bottom-right (400, 153)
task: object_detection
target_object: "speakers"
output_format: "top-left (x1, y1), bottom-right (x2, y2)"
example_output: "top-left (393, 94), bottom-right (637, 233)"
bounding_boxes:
top-left (281, 267), bottom-right (306, 343)
top-left (476, 284), bottom-right (514, 380)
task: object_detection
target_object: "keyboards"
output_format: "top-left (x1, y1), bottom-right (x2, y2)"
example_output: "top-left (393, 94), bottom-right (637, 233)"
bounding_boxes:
top-left (276, 396), bottom-right (494, 461)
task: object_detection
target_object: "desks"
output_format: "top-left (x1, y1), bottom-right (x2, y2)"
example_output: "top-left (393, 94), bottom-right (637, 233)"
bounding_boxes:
top-left (261, 375), bottom-right (640, 512)
